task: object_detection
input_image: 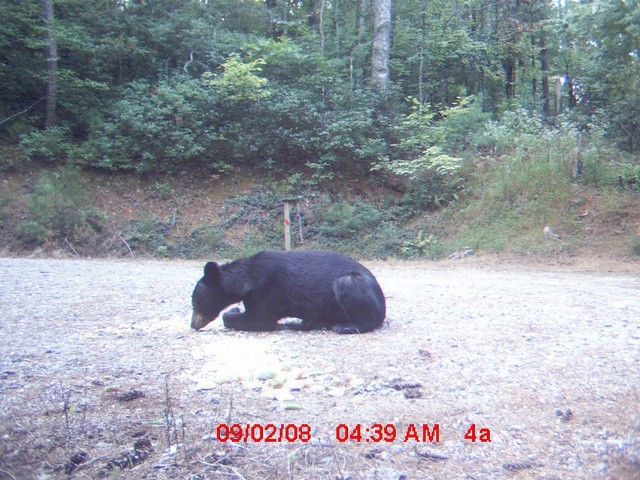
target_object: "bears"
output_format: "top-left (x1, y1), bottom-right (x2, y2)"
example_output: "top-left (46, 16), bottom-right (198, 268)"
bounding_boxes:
top-left (191, 250), bottom-right (386, 334)
